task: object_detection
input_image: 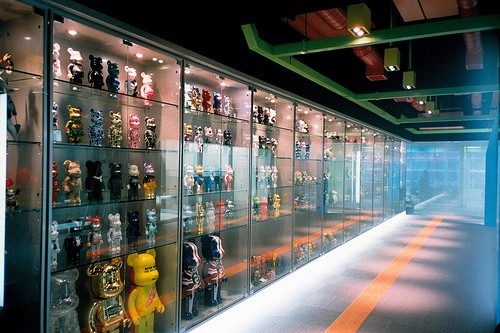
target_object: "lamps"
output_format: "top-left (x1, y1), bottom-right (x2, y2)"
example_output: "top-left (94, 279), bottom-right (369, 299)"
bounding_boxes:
top-left (346, 4), bottom-right (371, 38)
top-left (402, 40), bottom-right (415, 91)
top-left (383, 10), bottom-right (401, 72)
top-left (416, 96), bottom-right (428, 105)
top-left (425, 96), bottom-right (435, 114)
top-left (431, 96), bottom-right (440, 120)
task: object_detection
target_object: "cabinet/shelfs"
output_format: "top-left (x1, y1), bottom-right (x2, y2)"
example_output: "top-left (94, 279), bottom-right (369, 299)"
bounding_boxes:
top-left (0, 0), bottom-right (406, 333)
